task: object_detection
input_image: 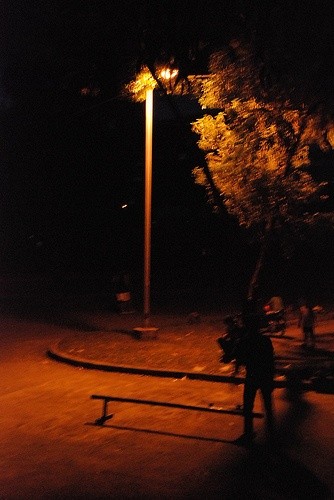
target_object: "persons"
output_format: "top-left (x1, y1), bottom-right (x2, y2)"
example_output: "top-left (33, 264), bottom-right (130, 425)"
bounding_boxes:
top-left (217, 315), bottom-right (239, 363)
top-left (267, 294), bottom-right (283, 312)
top-left (234, 299), bottom-right (277, 445)
top-left (298, 300), bottom-right (317, 350)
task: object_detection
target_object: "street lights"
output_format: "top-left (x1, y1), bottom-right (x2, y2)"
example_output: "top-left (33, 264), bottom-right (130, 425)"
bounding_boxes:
top-left (131, 45), bottom-right (182, 334)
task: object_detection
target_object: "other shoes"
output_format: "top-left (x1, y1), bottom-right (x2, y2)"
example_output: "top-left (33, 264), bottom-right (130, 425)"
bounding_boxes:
top-left (220, 355), bottom-right (231, 363)
top-left (234, 433), bottom-right (256, 446)
